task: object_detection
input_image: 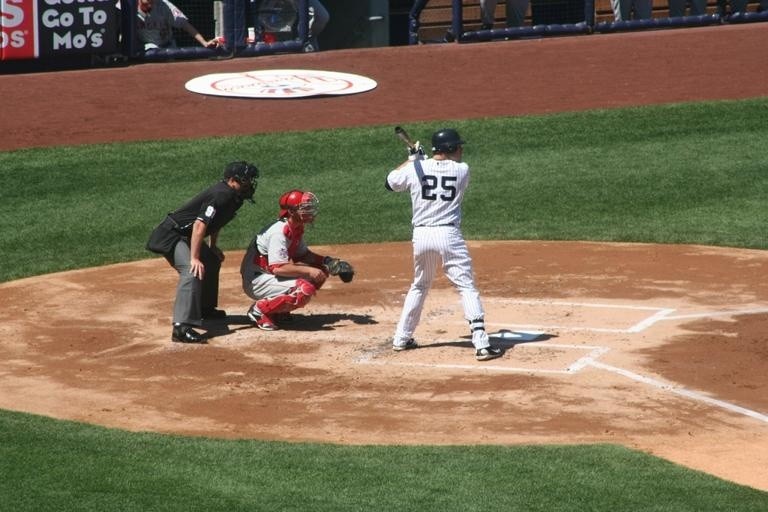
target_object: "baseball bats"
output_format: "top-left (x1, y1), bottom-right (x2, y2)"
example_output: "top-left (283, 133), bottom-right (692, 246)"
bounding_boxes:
top-left (395, 127), bottom-right (416, 151)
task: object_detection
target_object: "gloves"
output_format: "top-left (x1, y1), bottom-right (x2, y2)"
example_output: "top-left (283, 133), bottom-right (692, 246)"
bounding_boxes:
top-left (408, 140), bottom-right (428, 160)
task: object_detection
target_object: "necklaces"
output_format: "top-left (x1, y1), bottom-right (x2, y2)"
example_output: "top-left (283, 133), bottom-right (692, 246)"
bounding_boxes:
top-left (301, 1), bottom-right (330, 55)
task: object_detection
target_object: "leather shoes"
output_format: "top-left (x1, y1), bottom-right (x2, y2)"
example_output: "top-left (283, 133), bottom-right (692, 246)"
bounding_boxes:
top-left (172, 325), bottom-right (206, 342)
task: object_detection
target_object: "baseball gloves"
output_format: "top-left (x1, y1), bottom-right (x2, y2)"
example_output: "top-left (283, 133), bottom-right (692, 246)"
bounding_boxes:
top-left (324, 257), bottom-right (354, 282)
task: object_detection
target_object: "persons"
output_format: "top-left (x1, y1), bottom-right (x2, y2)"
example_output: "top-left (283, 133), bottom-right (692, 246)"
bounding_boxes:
top-left (667, 0), bottom-right (708, 17)
top-left (383, 129), bottom-right (504, 362)
top-left (718, 1), bottom-right (747, 17)
top-left (142, 162), bottom-right (258, 344)
top-left (479, 1), bottom-right (531, 43)
top-left (115, 1), bottom-right (220, 52)
top-left (240, 188), bottom-right (355, 331)
top-left (612, 1), bottom-right (654, 23)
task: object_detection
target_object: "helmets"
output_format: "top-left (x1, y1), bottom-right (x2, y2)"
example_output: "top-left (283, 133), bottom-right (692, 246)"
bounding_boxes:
top-left (279, 191), bottom-right (318, 222)
top-left (432, 129), bottom-right (465, 153)
top-left (224, 162), bottom-right (258, 199)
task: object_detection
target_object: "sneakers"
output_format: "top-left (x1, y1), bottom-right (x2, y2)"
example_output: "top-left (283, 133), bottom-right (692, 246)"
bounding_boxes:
top-left (392, 338), bottom-right (416, 350)
top-left (475, 346), bottom-right (501, 360)
top-left (247, 305), bottom-right (274, 330)
